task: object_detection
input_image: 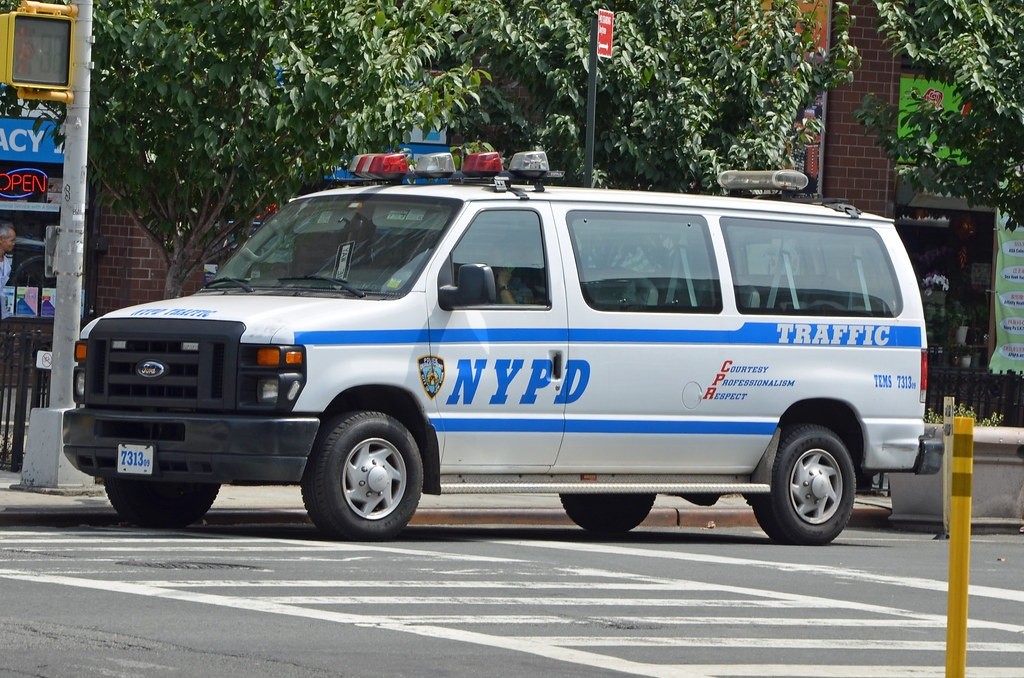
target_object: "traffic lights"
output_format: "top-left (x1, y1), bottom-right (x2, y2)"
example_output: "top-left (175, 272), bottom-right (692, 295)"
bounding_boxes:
top-left (0, 10), bottom-right (75, 92)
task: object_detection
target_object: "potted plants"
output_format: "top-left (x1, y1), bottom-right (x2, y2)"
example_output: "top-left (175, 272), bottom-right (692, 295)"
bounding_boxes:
top-left (923, 306), bottom-right (990, 368)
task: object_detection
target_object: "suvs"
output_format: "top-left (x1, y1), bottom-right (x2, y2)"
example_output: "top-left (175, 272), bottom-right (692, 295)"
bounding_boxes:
top-left (61, 147), bottom-right (929, 546)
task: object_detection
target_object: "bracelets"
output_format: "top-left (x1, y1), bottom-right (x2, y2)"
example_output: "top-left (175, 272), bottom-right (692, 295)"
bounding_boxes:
top-left (499, 285), bottom-right (509, 291)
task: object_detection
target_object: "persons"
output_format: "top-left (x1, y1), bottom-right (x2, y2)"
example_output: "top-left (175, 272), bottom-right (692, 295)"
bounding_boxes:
top-left (0, 224), bottom-right (18, 285)
top-left (491, 265), bottom-right (534, 305)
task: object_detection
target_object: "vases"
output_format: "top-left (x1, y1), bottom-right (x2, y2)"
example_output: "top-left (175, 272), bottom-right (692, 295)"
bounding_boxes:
top-left (935, 291), bottom-right (945, 318)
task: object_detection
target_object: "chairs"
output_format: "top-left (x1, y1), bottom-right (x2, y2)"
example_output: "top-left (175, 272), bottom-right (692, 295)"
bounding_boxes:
top-left (734, 283), bottom-right (761, 307)
top-left (583, 268), bottom-right (660, 306)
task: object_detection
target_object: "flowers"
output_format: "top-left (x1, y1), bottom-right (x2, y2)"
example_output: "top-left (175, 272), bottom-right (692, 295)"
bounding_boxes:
top-left (922, 271), bottom-right (950, 296)
